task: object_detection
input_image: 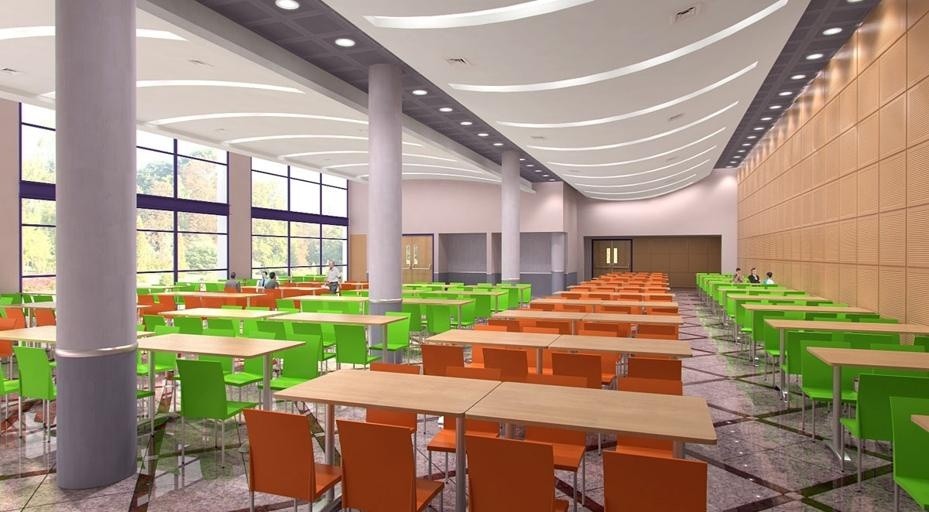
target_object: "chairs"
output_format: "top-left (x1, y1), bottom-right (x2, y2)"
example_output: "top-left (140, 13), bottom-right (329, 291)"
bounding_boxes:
top-left (0, 292), bottom-right (55, 445)
top-left (697, 272), bottom-right (925, 511)
top-left (138, 271), bottom-right (718, 510)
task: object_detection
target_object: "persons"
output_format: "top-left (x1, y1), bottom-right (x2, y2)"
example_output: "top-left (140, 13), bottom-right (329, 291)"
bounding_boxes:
top-left (225, 272), bottom-right (240, 289)
top-left (764, 272), bottom-right (774, 284)
top-left (257, 272), bottom-right (268, 287)
top-left (733, 267), bottom-right (744, 284)
top-left (327, 260), bottom-right (343, 294)
top-left (264, 272), bottom-right (279, 289)
top-left (748, 268), bottom-right (759, 283)
